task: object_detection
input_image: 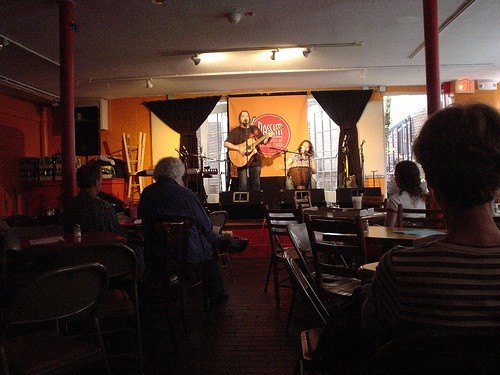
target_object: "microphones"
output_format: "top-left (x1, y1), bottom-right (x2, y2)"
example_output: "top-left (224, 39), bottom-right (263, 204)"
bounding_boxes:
top-left (342, 134), bottom-right (348, 148)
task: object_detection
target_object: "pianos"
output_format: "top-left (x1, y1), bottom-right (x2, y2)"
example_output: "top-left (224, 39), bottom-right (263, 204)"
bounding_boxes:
top-left (138, 165), bottom-right (220, 204)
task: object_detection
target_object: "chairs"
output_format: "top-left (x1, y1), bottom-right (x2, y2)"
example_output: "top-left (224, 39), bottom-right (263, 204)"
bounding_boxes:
top-left (0, 201), bottom-right (236, 375)
top-left (262, 199), bottom-right (500, 375)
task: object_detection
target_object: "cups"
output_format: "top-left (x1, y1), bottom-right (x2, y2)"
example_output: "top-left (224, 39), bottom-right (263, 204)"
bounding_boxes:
top-left (352, 196), bottom-right (362, 210)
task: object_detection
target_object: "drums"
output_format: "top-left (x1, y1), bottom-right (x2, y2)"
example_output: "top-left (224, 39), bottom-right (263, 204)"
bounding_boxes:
top-left (287, 166), bottom-right (313, 190)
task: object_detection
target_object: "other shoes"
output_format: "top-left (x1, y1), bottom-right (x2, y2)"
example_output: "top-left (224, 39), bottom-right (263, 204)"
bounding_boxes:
top-left (209, 290), bottom-right (229, 304)
top-left (219, 238), bottom-right (249, 254)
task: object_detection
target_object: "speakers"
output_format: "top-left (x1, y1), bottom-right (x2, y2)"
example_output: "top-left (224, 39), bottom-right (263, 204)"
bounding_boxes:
top-left (282, 188), bottom-right (325, 209)
top-left (73, 106), bottom-right (101, 156)
top-left (218, 189), bottom-right (264, 219)
top-left (336, 187), bottom-right (382, 208)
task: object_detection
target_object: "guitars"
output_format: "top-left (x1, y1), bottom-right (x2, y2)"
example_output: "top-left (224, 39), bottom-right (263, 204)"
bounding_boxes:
top-left (228, 125), bottom-right (284, 169)
top-left (337, 147), bottom-right (358, 188)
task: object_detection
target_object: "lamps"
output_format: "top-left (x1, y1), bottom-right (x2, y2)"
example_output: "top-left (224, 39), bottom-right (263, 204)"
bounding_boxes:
top-left (303, 47), bottom-right (313, 58)
top-left (146, 77), bottom-right (154, 88)
top-left (3, 38), bottom-right (9, 46)
top-left (270, 48), bottom-right (279, 60)
top-left (191, 53), bottom-right (201, 65)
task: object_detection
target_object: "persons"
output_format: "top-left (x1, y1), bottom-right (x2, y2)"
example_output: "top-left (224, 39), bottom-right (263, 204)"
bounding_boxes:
top-left (61, 164), bottom-right (148, 274)
top-left (138, 157), bottom-right (249, 303)
top-left (360, 104), bottom-right (500, 343)
top-left (286, 140), bottom-right (317, 189)
top-left (224, 110), bottom-right (274, 203)
top-left (384, 161), bottom-right (426, 227)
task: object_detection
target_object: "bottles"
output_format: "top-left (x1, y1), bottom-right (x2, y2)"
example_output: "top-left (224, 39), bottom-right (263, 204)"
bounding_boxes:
top-left (47, 206), bottom-right (51, 215)
top-left (74, 224), bottom-right (81, 237)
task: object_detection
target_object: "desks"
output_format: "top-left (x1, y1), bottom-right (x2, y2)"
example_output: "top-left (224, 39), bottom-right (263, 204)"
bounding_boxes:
top-left (4, 231), bottom-right (127, 247)
top-left (322, 225), bottom-right (447, 256)
top-left (362, 211), bottom-right (387, 223)
